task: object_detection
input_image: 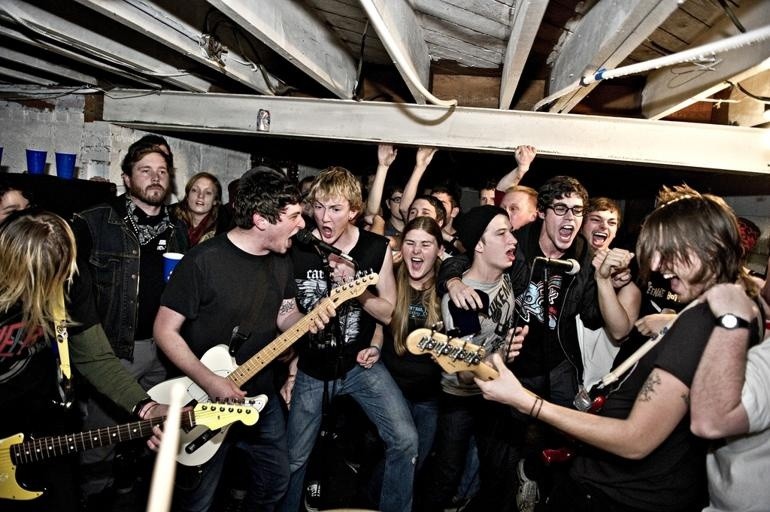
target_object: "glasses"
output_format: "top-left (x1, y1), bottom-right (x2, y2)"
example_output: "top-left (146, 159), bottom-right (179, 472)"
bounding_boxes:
top-left (389, 196), bottom-right (402, 204)
top-left (547, 202), bottom-right (587, 218)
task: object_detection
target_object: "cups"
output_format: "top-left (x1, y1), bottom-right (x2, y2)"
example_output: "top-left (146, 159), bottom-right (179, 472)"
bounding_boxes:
top-left (55, 150), bottom-right (77, 179)
top-left (162, 252), bottom-right (186, 283)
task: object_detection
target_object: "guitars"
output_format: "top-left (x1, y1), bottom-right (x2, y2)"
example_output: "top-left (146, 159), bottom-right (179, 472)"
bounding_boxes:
top-left (406, 323), bottom-right (606, 467)
top-left (0, 398), bottom-right (259, 501)
top-left (146, 267), bottom-right (379, 467)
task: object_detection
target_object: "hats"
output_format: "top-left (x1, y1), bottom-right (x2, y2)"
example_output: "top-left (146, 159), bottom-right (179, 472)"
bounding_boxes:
top-left (459, 203), bottom-right (510, 253)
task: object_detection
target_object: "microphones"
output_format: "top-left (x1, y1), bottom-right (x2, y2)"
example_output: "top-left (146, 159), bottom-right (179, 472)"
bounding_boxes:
top-left (296, 228), bottom-right (357, 265)
top-left (536, 255), bottom-right (582, 276)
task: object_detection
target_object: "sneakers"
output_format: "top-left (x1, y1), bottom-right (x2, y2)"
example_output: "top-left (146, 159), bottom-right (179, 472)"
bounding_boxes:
top-left (304, 479), bottom-right (323, 512)
top-left (115, 468), bottom-right (135, 495)
top-left (514, 457), bottom-right (541, 512)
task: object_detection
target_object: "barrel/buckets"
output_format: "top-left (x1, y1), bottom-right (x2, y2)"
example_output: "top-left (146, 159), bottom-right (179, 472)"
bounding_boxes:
top-left (55, 153), bottom-right (77, 178)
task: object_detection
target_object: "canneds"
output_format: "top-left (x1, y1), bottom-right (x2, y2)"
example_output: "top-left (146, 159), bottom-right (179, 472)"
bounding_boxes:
top-left (257, 110), bottom-right (269, 131)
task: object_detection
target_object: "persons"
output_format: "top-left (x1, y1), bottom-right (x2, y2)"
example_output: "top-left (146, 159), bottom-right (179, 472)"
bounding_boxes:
top-left (1, 135), bottom-right (770, 512)
